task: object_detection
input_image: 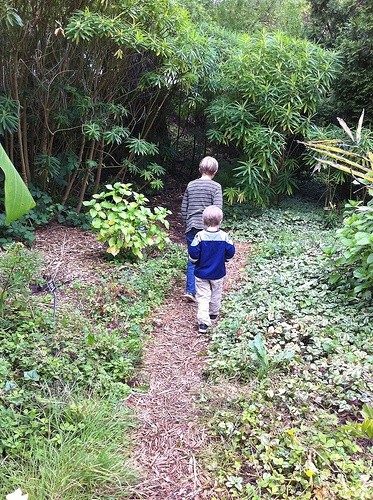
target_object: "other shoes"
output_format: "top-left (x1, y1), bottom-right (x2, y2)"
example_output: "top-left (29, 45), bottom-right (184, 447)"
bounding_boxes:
top-left (198, 324), bottom-right (208, 332)
top-left (184, 292), bottom-right (195, 302)
top-left (210, 315), bottom-right (218, 320)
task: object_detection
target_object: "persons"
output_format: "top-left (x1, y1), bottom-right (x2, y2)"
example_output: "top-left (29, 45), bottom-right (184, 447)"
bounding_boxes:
top-left (188, 204), bottom-right (235, 334)
top-left (181, 156), bottom-right (223, 303)
